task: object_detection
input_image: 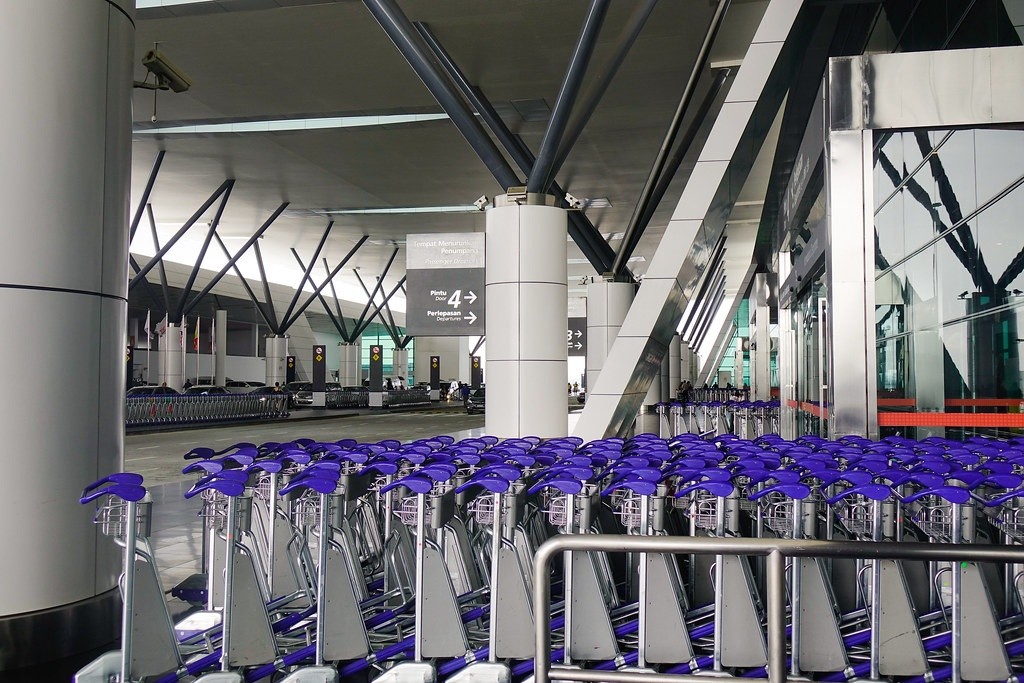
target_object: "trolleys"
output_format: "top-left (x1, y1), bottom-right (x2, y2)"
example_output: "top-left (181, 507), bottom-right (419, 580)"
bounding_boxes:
top-left (657, 400), bottom-right (781, 439)
top-left (72, 438), bottom-right (1023, 683)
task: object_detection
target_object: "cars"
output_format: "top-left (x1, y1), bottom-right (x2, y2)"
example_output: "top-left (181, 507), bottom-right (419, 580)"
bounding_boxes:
top-left (440, 380), bottom-right (485, 415)
top-left (412, 381), bottom-right (432, 391)
top-left (127, 384), bottom-right (234, 398)
top-left (227, 380), bottom-right (370, 408)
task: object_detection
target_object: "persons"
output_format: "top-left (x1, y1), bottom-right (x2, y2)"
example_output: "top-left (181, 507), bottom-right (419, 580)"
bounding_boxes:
top-left (162, 379), bottom-right (287, 413)
top-left (385, 378), bottom-right (471, 408)
top-left (568, 382), bottom-right (579, 395)
top-left (678, 380), bottom-right (748, 403)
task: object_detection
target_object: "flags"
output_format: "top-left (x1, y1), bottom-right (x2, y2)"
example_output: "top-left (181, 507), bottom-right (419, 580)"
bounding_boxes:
top-left (144, 309), bottom-right (216, 353)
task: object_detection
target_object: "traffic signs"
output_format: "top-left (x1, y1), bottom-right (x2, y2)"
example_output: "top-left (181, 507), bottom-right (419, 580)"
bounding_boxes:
top-left (406, 267), bottom-right (485, 337)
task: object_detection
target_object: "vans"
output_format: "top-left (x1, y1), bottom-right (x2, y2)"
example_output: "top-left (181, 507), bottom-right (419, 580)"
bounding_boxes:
top-left (364, 375), bottom-right (408, 390)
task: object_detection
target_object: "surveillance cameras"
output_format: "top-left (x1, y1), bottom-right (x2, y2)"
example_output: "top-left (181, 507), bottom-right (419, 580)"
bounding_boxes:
top-left (141, 49), bottom-right (193, 95)
top-left (565, 193), bottom-right (581, 207)
top-left (474, 194), bottom-right (489, 207)
